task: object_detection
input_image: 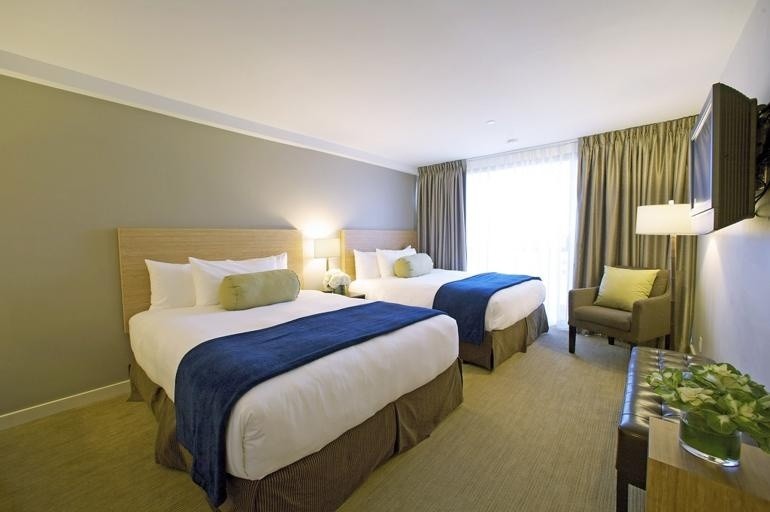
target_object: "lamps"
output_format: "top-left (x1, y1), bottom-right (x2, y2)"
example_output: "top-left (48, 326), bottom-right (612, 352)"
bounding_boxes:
top-left (636, 199), bottom-right (692, 348)
top-left (311, 238), bottom-right (340, 269)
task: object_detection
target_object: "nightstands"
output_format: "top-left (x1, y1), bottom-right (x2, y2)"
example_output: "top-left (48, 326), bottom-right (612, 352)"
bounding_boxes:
top-left (346, 292), bottom-right (365, 298)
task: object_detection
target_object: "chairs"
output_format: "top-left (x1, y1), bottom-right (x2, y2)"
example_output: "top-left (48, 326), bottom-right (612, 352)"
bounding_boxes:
top-left (568, 265), bottom-right (672, 356)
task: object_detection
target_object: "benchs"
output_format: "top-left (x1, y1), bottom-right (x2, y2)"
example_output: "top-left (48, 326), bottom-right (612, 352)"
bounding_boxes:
top-left (615, 344), bottom-right (716, 512)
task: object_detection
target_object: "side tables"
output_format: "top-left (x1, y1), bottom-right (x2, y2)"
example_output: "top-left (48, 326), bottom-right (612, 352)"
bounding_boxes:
top-left (645, 416), bottom-right (769, 512)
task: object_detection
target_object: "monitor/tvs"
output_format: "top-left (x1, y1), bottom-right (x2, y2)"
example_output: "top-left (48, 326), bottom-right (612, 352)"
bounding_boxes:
top-left (687, 81), bottom-right (758, 236)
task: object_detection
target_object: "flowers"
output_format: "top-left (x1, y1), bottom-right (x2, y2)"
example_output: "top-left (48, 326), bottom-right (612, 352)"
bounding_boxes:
top-left (648, 361), bottom-right (770, 452)
top-left (325, 269), bottom-right (352, 289)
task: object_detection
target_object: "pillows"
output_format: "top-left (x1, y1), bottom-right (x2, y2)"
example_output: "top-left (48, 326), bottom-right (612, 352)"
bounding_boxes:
top-left (592, 264), bottom-right (659, 307)
top-left (352, 249), bottom-right (380, 280)
top-left (219, 268), bottom-right (301, 310)
top-left (396, 253), bottom-right (435, 279)
top-left (143, 259), bottom-right (195, 309)
top-left (374, 247), bottom-right (417, 278)
top-left (182, 252), bottom-right (287, 306)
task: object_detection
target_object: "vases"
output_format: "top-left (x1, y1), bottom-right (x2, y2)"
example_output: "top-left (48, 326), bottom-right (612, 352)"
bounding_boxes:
top-left (678, 409), bottom-right (744, 466)
top-left (333, 284), bottom-right (346, 295)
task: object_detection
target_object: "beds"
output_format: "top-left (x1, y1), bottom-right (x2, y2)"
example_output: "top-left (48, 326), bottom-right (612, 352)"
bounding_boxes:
top-left (112, 228), bottom-right (465, 512)
top-left (341, 230), bottom-right (550, 369)
